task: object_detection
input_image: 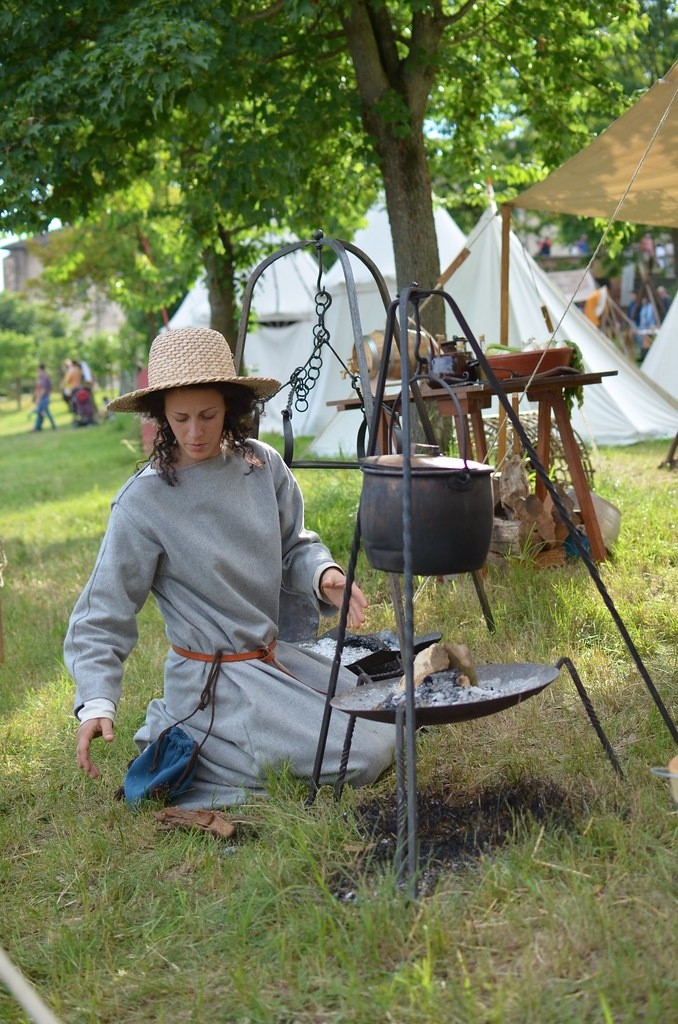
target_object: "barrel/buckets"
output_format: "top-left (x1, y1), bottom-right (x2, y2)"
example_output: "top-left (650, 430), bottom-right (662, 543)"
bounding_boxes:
top-left (351, 331), bottom-right (437, 378)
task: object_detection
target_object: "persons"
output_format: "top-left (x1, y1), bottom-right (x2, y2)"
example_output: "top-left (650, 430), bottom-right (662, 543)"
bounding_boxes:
top-left (59, 325), bottom-right (410, 812)
top-left (28, 230), bottom-right (674, 431)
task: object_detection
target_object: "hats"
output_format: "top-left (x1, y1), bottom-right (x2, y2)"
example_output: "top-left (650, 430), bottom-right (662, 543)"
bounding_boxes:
top-left (107, 327), bottom-right (282, 412)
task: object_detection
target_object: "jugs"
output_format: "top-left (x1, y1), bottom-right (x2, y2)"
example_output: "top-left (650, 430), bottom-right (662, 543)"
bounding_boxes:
top-left (428, 351), bottom-right (480, 389)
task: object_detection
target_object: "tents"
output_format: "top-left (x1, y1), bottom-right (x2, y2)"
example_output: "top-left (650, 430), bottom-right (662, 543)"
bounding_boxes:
top-left (157, 184), bottom-right (678, 460)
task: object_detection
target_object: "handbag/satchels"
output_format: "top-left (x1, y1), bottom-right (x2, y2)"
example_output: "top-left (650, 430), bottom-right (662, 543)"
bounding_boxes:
top-left (114, 650), bottom-right (222, 809)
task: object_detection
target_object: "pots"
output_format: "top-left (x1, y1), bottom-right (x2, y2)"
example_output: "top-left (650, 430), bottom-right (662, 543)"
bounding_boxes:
top-left (359, 375), bottom-right (495, 576)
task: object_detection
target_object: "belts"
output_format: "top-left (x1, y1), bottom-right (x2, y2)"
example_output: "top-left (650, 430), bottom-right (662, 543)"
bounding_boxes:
top-left (172, 641), bottom-right (331, 694)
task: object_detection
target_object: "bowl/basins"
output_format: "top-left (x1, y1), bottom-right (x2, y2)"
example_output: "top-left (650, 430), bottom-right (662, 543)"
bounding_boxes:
top-left (485, 348), bottom-right (574, 379)
top-left (569, 487), bottom-right (624, 542)
